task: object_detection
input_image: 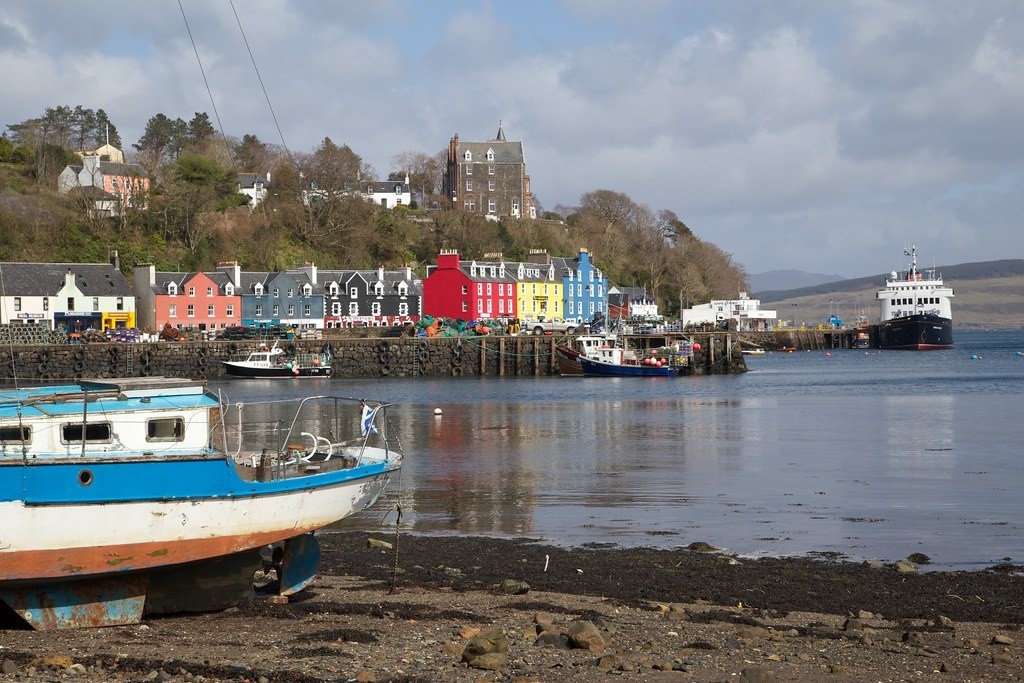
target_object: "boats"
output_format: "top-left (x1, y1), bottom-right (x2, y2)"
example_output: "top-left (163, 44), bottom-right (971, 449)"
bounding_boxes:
top-left (741, 348), bottom-right (766, 355)
top-left (555, 298), bottom-right (669, 378)
top-left (874, 243), bottom-right (956, 350)
top-left (221, 335), bottom-right (334, 382)
top-left (0, 376), bottom-right (230, 455)
top-left (578, 348), bottom-right (685, 377)
top-left (777, 348), bottom-right (796, 351)
top-left (0, 396), bottom-right (404, 632)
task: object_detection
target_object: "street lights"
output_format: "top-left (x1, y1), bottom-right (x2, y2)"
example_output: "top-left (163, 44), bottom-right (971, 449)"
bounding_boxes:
top-left (836, 298), bottom-right (840, 320)
top-left (791, 303), bottom-right (798, 328)
top-left (828, 298), bottom-right (833, 322)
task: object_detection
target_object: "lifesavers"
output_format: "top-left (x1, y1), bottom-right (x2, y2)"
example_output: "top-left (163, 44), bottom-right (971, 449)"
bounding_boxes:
top-left (199, 357), bottom-right (208, 365)
top-left (379, 341), bottom-right (390, 352)
top-left (199, 375), bottom-right (206, 379)
top-left (419, 341), bottom-right (428, 350)
top-left (285, 343), bottom-right (295, 355)
top-left (111, 346), bottom-right (119, 373)
top-left (380, 364), bottom-right (392, 376)
top-left (142, 365), bottom-right (153, 375)
top-left (420, 365), bottom-right (429, 373)
top-left (451, 367), bottom-right (464, 377)
top-left (419, 351), bottom-right (430, 362)
top-left (9, 351), bottom-right (19, 377)
top-left (452, 356), bottom-right (464, 366)
top-left (200, 347), bottom-right (209, 356)
top-left (228, 344), bottom-right (238, 354)
top-left (322, 343), bottom-right (334, 354)
top-left (453, 344), bottom-right (464, 355)
top-left (142, 351), bottom-right (154, 362)
top-left (380, 352), bottom-right (391, 364)
top-left (37, 348), bottom-right (53, 385)
top-left (73, 348), bottom-right (85, 372)
top-left (259, 347), bottom-right (269, 352)
top-left (198, 366), bottom-right (208, 375)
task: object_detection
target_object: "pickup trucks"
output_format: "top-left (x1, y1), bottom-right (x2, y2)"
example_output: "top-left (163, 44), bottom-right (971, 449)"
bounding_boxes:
top-left (525, 316), bottom-right (581, 337)
